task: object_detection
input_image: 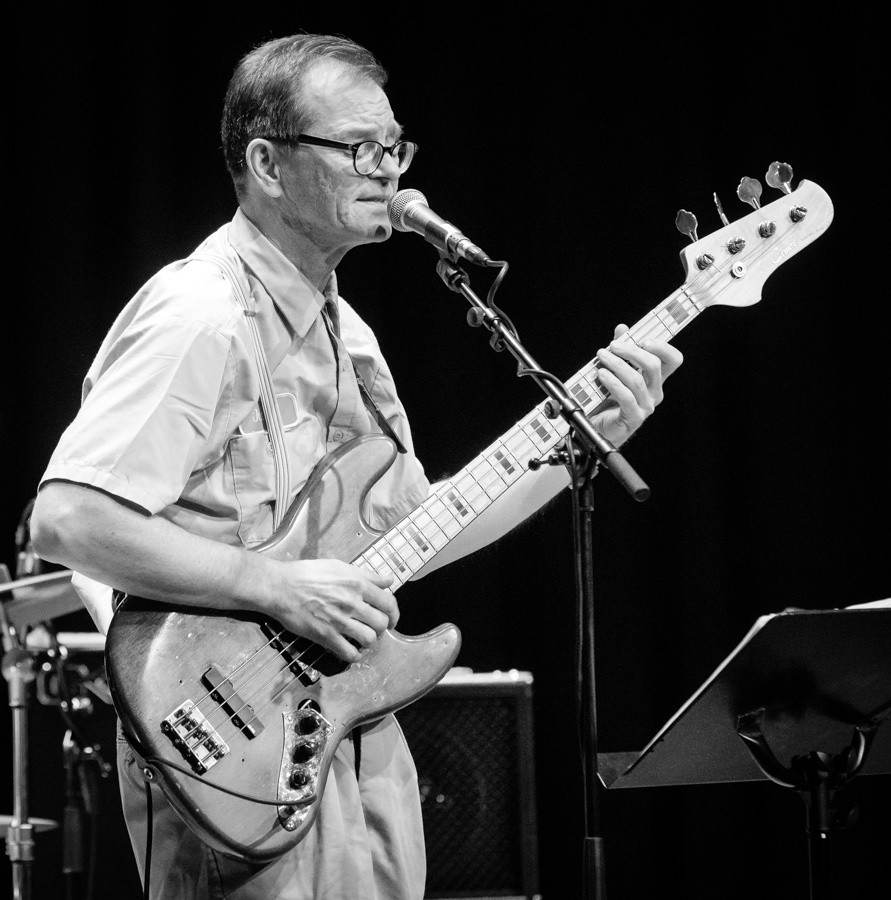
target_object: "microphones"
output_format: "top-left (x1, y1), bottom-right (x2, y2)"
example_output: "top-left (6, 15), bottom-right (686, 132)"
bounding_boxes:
top-left (387, 188), bottom-right (489, 266)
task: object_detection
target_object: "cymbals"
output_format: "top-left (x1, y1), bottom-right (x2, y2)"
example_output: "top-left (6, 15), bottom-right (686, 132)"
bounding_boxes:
top-left (0, 568), bottom-right (85, 629)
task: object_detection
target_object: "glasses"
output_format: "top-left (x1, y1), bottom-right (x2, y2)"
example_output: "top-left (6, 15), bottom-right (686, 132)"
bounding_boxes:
top-left (296, 133), bottom-right (418, 177)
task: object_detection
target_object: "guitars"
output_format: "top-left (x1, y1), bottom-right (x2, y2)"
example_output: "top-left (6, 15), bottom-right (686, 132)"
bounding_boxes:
top-left (104, 158), bottom-right (836, 866)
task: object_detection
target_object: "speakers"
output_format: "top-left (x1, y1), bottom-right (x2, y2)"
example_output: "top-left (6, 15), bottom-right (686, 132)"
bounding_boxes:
top-left (394, 667), bottom-right (540, 900)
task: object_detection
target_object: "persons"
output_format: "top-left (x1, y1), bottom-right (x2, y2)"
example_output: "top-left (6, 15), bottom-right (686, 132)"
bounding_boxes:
top-left (28, 34), bottom-right (684, 900)
